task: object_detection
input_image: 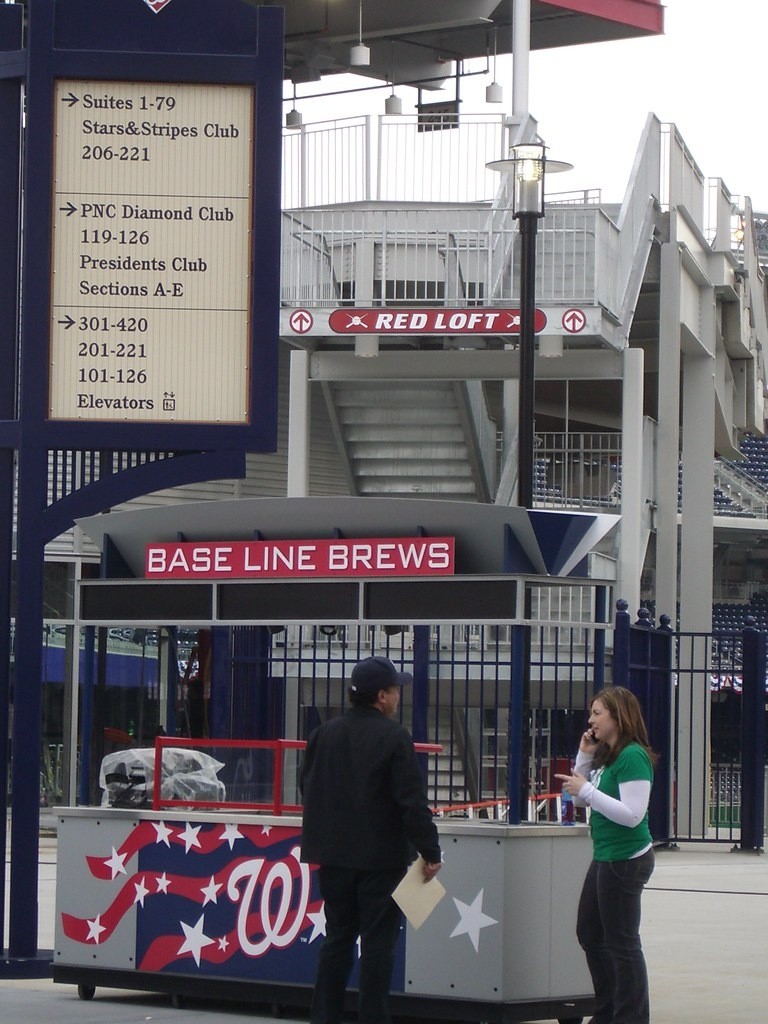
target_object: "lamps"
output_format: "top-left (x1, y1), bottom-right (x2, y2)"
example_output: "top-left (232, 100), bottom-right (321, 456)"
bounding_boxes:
top-left (384, 40), bottom-right (403, 114)
top-left (486, 28), bottom-right (504, 103)
top-left (285, 83), bottom-right (303, 131)
top-left (348, 0), bottom-right (370, 67)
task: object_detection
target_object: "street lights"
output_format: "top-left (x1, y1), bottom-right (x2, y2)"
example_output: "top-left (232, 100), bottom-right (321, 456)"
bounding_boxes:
top-left (481, 133), bottom-right (575, 822)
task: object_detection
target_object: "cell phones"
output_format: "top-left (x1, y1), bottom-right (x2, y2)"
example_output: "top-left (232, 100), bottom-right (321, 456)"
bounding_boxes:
top-left (589, 731), bottom-right (600, 745)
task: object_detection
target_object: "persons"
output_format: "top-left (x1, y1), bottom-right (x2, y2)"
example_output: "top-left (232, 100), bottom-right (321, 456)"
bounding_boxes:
top-left (554, 686), bottom-right (655, 1024)
top-left (298, 656), bottom-right (442, 1024)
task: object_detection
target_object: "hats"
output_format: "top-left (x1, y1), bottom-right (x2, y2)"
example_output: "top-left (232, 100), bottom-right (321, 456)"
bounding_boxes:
top-left (351, 657), bottom-right (413, 702)
